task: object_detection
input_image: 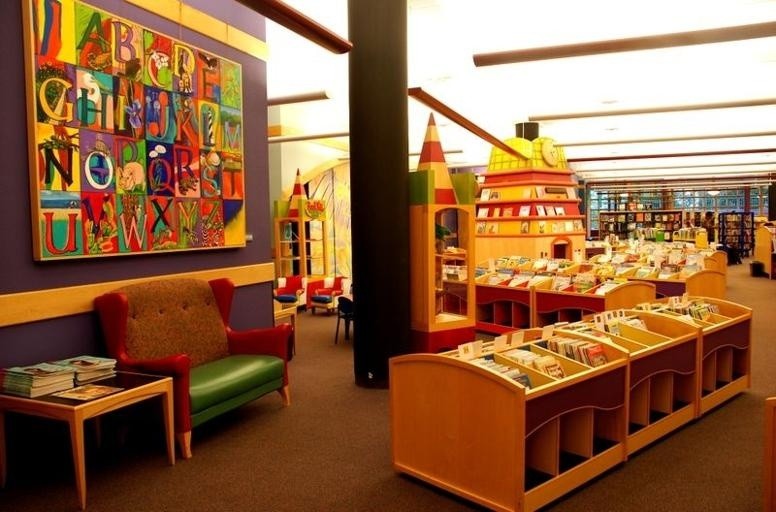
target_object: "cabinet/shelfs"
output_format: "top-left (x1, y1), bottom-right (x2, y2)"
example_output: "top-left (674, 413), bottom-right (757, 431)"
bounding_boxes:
top-left (476, 169), bottom-right (585, 269)
top-left (274, 168), bottom-right (328, 277)
top-left (599, 209), bottom-right (755, 266)
top-left (389, 296), bottom-right (753, 512)
top-left (752, 227), bottom-right (775, 280)
top-left (408, 112), bottom-right (475, 353)
top-left (476, 241), bottom-right (731, 333)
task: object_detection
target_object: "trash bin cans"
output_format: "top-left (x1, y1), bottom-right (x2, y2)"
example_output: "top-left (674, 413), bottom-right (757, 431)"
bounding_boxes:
top-left (749, 261), bottom-right (762, 277)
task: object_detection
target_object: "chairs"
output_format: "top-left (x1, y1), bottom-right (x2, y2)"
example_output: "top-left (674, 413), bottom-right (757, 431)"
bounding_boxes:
top-left (306, 277), bottom-right (352, 316)
top-left (335, 297), bottom-right (353, 344)
top-left (273, 276), bottom-right (307, 314)
top-left (94, 276), bottom-right (296, 459)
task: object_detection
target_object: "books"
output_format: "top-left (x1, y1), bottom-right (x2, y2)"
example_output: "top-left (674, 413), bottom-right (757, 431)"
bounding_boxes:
top-left (503, 348), bottom-right (566, 379)
top-left (572, 263), bottom-right (659, 296)
top-left (538, 220), bottom-right (545, 233)
top-left (545, 336), bottom-right (608, 368)
top-left (584, 310), bottom-right (648, 344)
top-left (474, 255), bottom-right (534, 288)
top-left (458, 327), bottom-right (546, 360)
top-left (674, 300), bottom-right (719, 323)
top-left (720, 214), bottom-right (751, 251)
top-left (470, 358), bottom-right (532, 392)
top-left (589, 242), bottom-right (720, 280)
top-left (478, 205), bottom-right (567, 217)
top-left (636, 302), bottom-right (661, 310)
top-left (521, 220), bottom-right (530, 233)
top-left (442, 245), bottom-right (468, 281)
top-left (600, 214), bottom-right (682, 241)
top-left (0, 354), bottom-right (117, 399)
top-left (685, 212), bottom-right (715, 244)
top-left (529, 257), bottom-right (577, 291)
top-left (476, 221), bottom-right (499, 234)
top-left (552, 220), bottom-right (583, 233)
